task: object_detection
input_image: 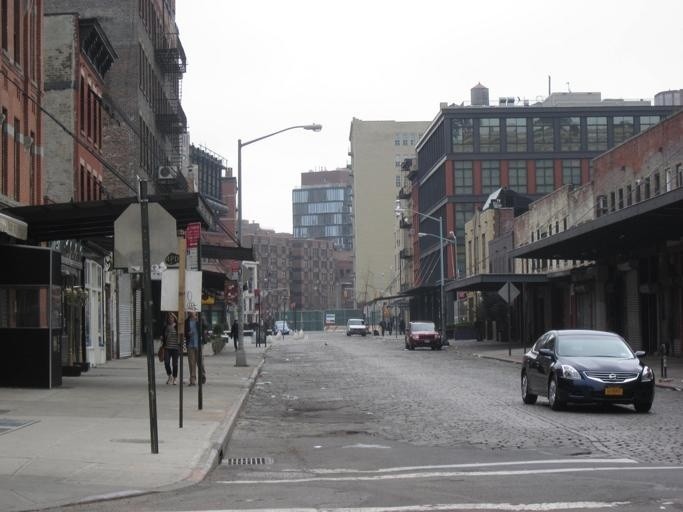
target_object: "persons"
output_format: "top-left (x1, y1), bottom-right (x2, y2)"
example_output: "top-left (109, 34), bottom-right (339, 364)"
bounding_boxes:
top-left (229, 319), bottom-right (238, 352)
top-left (185, 310), bottom-right (206, 387)
top-left (158, 311), bottom-right (180, 385)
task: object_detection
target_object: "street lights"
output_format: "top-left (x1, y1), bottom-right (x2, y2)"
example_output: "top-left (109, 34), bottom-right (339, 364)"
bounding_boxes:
top-left (395, 208), bottom-right (449, 343)
top-left (419, 230), bottom-right (458, 276)
top-left (231, 122), bottom-right (324, 365)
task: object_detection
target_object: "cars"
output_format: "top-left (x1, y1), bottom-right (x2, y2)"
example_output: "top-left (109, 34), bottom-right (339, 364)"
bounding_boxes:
top-left (274, 320), bottom-right (290, 334)
top-left (346, 319), bottom-right (367, 337)
top-left (520, 327), bottom-right (656, 415)
top-left (404, 321), bottom-right (443, 351)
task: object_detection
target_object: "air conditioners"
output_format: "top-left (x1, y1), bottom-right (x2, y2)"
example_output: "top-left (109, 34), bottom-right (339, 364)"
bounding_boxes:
top-left (157, 166), bottom-right (177, 179)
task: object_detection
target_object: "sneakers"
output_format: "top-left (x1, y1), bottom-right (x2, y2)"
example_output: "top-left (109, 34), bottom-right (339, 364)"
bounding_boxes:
top-left (189, 384), bottom-right (195, 386)
top-left (202, 371), bottom-right (206, 384)
top-left (166, 378), bottom-right (177, 385)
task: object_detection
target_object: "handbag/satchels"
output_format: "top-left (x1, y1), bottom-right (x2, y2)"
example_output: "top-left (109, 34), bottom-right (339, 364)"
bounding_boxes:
top-left (158, 348), bottom-right (165, 362)
